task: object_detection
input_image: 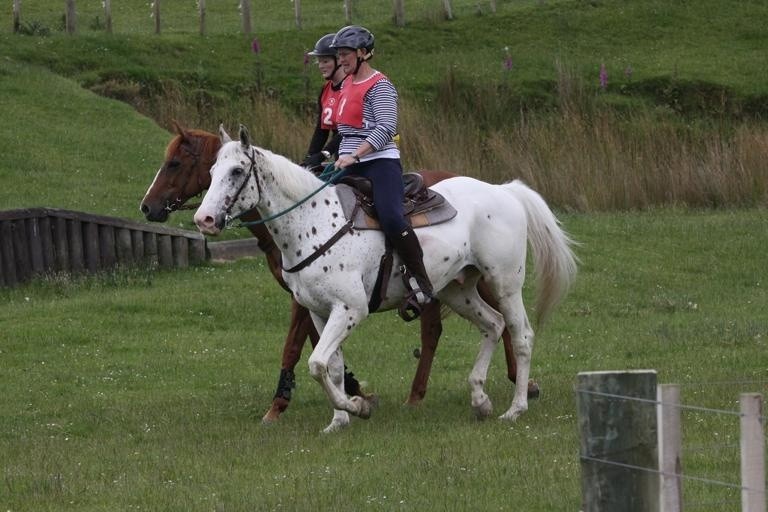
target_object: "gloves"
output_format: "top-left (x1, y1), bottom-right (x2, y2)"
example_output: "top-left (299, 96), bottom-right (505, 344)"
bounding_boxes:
top-left (299, 152), bottom-right (327, 168)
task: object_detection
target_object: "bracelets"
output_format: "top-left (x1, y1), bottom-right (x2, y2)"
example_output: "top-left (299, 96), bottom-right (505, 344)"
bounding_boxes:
top-left (320, 150), bottom-right (331, 158)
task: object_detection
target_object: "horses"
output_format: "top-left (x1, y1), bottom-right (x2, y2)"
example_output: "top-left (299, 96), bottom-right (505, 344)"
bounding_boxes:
top-left (140, 118), bottom-right (540, 425)
top-left (194, 122), bottom-right (586, 435)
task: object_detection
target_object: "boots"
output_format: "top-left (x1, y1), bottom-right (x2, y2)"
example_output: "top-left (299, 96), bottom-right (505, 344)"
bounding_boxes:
top-left (405, 259), bottom-right (434, 306)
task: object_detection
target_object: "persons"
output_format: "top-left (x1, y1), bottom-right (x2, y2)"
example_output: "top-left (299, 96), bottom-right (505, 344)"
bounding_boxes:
top-left (297, 32), bottom-right (347, 167)
top-left (325, 25), bottom-right (437, 322)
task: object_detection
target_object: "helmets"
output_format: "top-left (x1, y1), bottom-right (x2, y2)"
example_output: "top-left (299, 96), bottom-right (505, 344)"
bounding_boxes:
top-left (307, 25), bottom-right (375, 56)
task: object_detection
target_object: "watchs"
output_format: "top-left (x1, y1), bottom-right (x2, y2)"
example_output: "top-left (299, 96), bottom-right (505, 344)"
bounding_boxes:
top-left (350, 152), bottom-right (361, 162)
